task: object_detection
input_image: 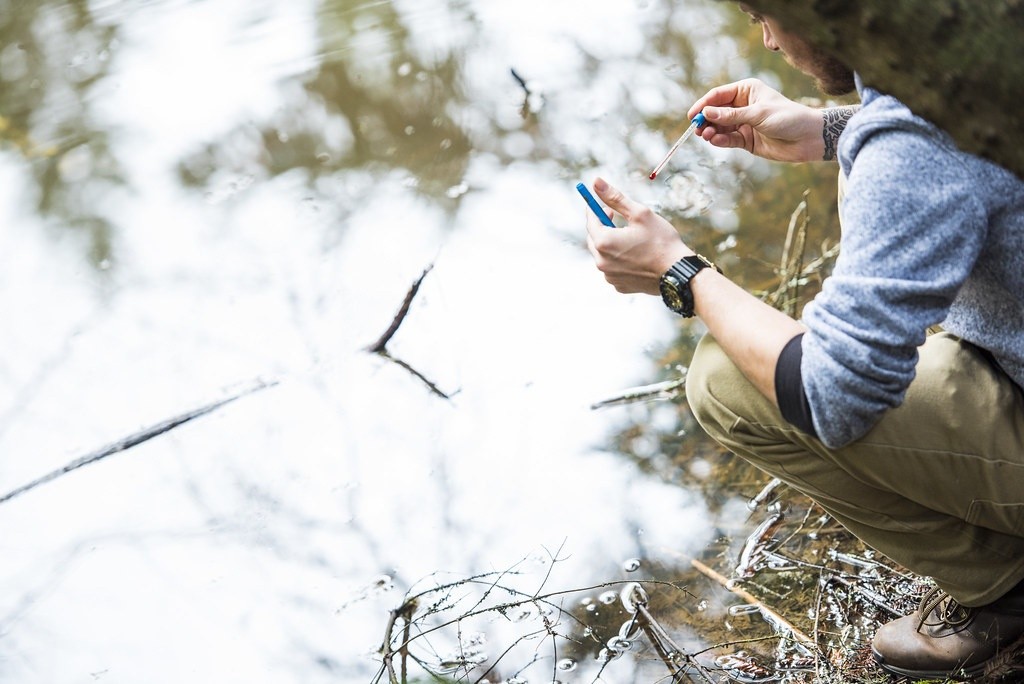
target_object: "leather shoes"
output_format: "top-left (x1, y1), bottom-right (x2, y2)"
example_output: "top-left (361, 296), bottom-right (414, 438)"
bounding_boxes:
top-left (871, 580), bottom-right (1024, 678)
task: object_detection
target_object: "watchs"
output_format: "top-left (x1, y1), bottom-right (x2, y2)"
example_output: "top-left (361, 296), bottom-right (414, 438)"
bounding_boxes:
top-left (658, 254), bottom-right (723, 317)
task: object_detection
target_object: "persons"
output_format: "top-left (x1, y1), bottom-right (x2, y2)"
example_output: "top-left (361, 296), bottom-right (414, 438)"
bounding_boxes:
top-left (583, 1), bottom-right (1023, 684)
